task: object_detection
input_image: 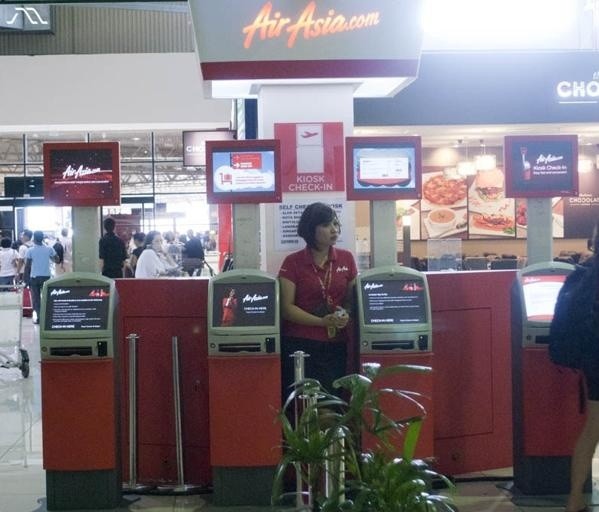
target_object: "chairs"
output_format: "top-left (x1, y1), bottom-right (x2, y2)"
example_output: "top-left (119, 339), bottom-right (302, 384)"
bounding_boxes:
top-left (226, 258), bottom-right (234, 270)
top-left (554, 256), bottom-right (575, 264)
top-left (491, 259), bottom-right (518, 269)
top-left (463, 257), bottom-right (487, 270)
top-left (440, 254), bottom-right (455, 268)
top-left (484, 253), bottom-right (496, 257)
top-left (222, 255), bottom-right (230, 272)
top-left (560, 250), bottom-right (579, 262)
top-left (502, 255), bottom-right (517, 268)
top-left (407, 257), bottom-right (421, 270)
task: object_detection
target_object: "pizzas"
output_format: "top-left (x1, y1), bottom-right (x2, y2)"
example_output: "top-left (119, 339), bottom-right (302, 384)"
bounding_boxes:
top-left (423, 175), bottom-right (467, 204)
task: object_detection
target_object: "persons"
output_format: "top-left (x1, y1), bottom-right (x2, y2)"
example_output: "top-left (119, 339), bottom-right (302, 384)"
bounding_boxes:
top-left (163, 233), bottom-right (184, 266)
top-left (57, 228), bottom-right (74, 275)
top-left (24, 231), bottom-right (61, 324)
top-left (132, 231), bottom-right (176, 278)
top-left (12, 231), bottom-right (24, 249)
top-left (185, 229), bottom-right (206, 278)
top-left (132, 231), bottom-right (149, 271)
top-left (546, 222), bottom-right (599, 512)
top-left (14, 230), bottom-right (33, 284)
top-left (96, 220), bottom-right (129, 280)
top-left (278, 202), bottom-right (357, 407)
top-left (0, 238), bottom-right (21, 294)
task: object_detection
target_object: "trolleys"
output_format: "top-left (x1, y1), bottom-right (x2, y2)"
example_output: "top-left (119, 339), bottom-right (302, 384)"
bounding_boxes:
top-left (0, 284), bottom-right (29, 378)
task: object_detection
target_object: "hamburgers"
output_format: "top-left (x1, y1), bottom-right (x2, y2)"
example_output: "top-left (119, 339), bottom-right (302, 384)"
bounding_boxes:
top-left (474, 167), bottom-right (505, 200)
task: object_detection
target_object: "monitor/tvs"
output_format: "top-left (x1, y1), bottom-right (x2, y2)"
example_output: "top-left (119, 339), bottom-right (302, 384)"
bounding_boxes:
top-left (522, 274), bottom-right (571, 322)
top-left (182, 129), bottom-right (238, 168)
top-left (213, 283), bottom-right (277, 328)
top-left (44, 286), bottom-right (108, 331)
top-left (360, 280), bottom-right (427, 325)
